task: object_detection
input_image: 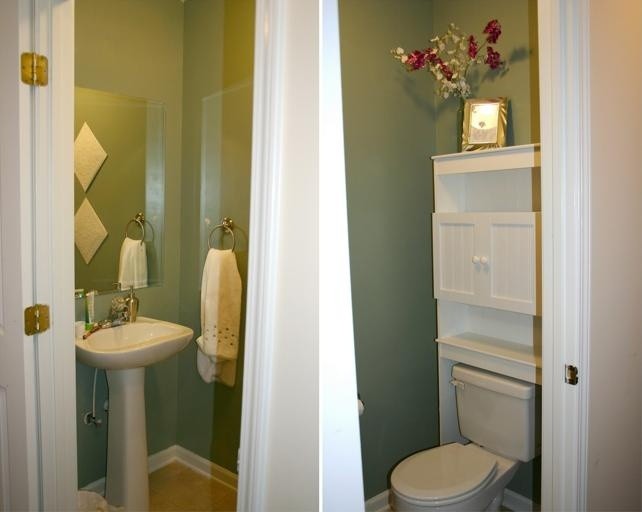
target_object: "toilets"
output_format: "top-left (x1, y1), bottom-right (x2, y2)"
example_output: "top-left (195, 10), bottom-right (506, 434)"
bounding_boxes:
top-left (389, 364), bottom-right (536, 512)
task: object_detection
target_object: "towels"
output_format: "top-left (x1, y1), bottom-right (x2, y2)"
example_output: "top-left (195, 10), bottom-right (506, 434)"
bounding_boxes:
top-left (196, 248), bottom-right (243, 388)
top-left (118, 237), bottom-right (148, 291)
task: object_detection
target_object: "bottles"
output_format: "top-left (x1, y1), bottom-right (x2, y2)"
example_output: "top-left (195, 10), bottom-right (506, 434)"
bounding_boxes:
top-left (75, 288), bottom-right (86, 339)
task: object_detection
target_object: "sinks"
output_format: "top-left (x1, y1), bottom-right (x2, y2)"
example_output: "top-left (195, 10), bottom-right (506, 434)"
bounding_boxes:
top-left (75, 318), bottom-right (194, 368)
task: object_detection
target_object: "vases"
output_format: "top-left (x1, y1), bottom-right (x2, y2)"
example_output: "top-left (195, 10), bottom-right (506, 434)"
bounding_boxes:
top-left (457, 97), bottom-right (465, 152)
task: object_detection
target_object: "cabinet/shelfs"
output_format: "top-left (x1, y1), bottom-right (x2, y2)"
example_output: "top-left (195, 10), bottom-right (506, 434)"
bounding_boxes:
top-left (433, 212), bottom-right (542, 316)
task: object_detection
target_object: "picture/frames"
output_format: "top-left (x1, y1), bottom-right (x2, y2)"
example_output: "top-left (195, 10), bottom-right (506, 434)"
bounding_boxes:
top-left (461, 97), bottom-right (508, 152)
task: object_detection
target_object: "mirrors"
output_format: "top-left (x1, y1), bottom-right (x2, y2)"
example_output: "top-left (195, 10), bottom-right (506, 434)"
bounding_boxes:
top-left (74, 87), bottom-right (165, 300)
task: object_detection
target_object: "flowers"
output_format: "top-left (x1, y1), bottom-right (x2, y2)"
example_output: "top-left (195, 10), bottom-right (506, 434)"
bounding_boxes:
top-left (390, 19), bottom-right (502, 100)
